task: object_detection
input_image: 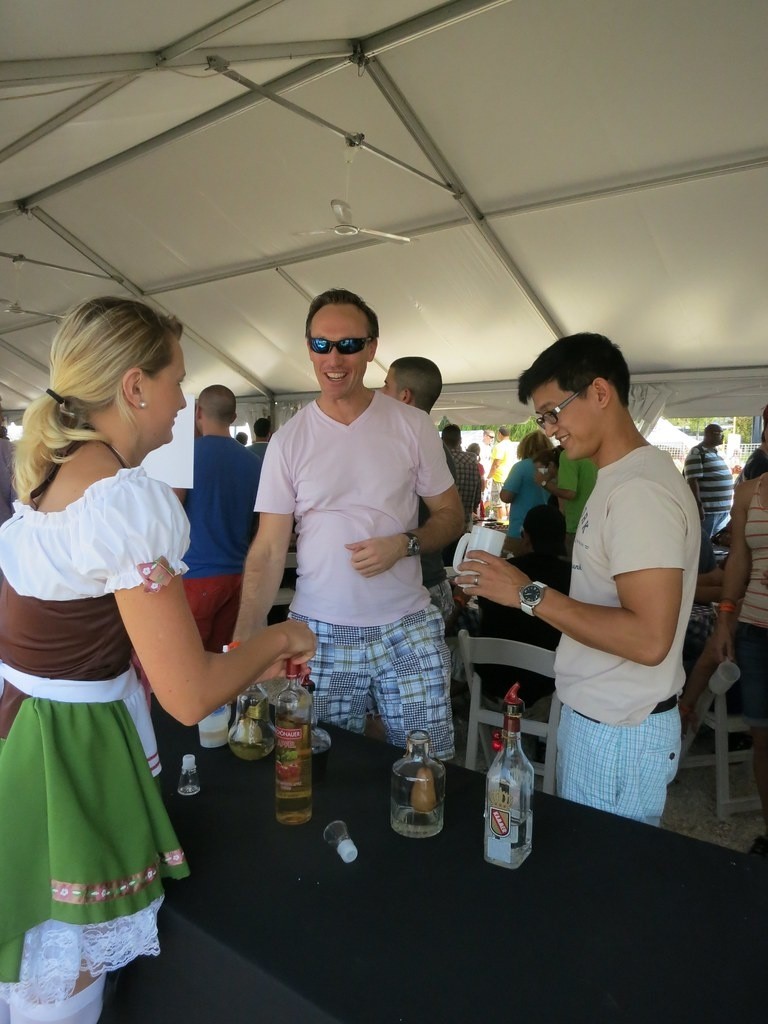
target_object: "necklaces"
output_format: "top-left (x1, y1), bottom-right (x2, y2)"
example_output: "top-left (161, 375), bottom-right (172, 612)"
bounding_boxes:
top-left (112, 446), bottom-right (132, 468)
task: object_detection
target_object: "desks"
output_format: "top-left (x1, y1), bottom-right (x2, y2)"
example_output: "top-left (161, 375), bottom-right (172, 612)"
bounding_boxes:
top-left (687, 543), bottom-right (732, 647)
top-left (94, 696), bottom-right (768, 1024)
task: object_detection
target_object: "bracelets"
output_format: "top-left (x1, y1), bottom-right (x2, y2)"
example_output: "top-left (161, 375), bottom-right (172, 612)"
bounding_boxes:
top-left (720, 598), bottom-right (737, 612)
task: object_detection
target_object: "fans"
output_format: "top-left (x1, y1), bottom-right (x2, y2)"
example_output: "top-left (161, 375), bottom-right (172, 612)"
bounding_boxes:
top-left (0, 260), bottom-right (67, 320)
top-left (288, 148), bottom-right (411, 246)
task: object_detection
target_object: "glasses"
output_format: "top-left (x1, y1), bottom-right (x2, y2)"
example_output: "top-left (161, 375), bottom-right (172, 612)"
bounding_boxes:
top-left (487, 435), bottom-right (495, 439)
top-left (308, 335), bottom-right (375, 354)
top-left (536, 375), bottom-right (608, 430)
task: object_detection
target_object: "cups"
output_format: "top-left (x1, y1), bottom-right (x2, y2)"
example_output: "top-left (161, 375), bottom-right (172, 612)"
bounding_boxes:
top-left (451, 526), bottom-right (504, 591)
top-left (707, 656), bottom-right (741, 695)
top-left (536, 466), bottom-right (549, 474)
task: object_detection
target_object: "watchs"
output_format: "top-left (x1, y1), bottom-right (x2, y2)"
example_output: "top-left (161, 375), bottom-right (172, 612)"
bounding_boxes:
top-left (403, 533), bottom-right (420, 556)
top-left (519, 581), bottom-right (548, 617)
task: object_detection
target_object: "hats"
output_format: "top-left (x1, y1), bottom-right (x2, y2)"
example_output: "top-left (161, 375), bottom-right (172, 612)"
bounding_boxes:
top-left (523, 504), bottom-right (568, 556)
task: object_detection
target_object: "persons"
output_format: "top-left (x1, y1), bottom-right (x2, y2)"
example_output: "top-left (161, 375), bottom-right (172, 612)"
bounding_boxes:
top-left (0, 296), bottom-right (316, 1024)
top-left (455, 332), bottom-right (701, 826)
top-left (381, 356), bottom-right (597, 715)
top-left (686, 424), bottom-right (768, 538)
top-left (680, 552), bottom-right (753, 754)
top-left (0, 396), bottom-right (18, 526)
top-left (174, 385), bottom-right (271, 654)
top-left (708, 403), bottom-right (768, 860)
top-left (232, 290), bottom-right (465, 761)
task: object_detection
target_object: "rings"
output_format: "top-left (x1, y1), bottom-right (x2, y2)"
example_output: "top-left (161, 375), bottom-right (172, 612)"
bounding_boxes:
top-left (474, 575), bottom-right (478, 585)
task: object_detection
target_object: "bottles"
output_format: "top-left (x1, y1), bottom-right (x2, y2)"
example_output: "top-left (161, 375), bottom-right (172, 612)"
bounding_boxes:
top-left (177, 755), bottom-right (200, 796)
top-left (389, 730), bottom-right (446, 838)
top-left (482, 682), bottom-right (533, 870)
top-left (197, 701), bottom-right (231, 748)
top-left (323, 819), bottom-right (358, 863)
top-left (273, 654), bottom-right (314, 824)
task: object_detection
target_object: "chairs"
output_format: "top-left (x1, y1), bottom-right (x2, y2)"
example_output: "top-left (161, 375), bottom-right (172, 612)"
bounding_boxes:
top-left (457, 629), bottom-right (563, 794)
top-left (679, 683), bottom-right (764, 820)
top-left (273, 552), bottom-right (298, 605)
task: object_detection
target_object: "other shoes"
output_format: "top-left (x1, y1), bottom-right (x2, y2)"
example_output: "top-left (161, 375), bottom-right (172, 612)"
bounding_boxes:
top-left (748, 835), bottom-right (768, 859)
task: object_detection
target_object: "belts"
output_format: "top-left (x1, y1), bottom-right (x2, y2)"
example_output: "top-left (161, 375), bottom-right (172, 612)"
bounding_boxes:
top-left (650, 694), bottom-right (679, 713)
